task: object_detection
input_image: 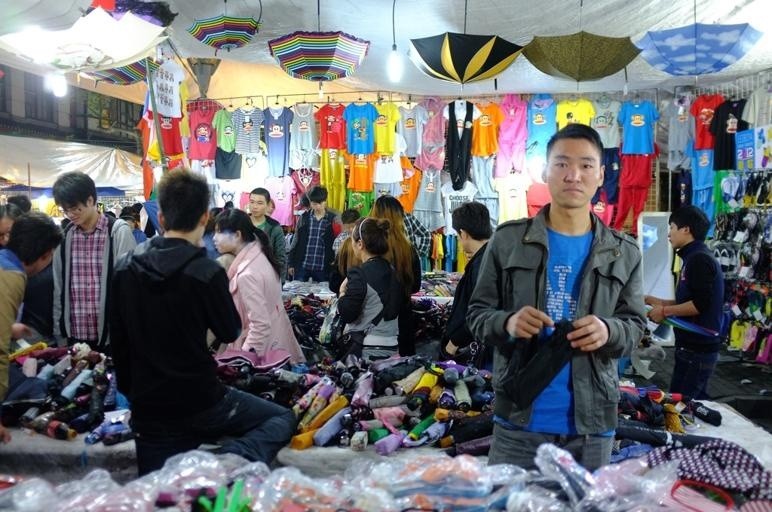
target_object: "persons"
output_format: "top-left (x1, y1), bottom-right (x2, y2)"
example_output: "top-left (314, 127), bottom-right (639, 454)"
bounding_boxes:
top-left (437, 201), bottom-right (493, 369)
top-left (1, 169), bottom-right (423, 474)
top-left (644, 204), bottom-right (725, 402)
top-left (464, 122), bottom-right (648, 474)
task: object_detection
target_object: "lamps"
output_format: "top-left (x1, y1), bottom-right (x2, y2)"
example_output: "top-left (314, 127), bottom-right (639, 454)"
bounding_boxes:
top-left (384, 1), bottom-right (404, 84)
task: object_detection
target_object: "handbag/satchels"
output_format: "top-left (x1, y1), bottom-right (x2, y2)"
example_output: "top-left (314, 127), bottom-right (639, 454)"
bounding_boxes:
top-left (318, 296), bottom-right (344, 345)
top-left (335, 330), bottom-right (367, 363)
top-left (438, 333), bottom-right (485, 369)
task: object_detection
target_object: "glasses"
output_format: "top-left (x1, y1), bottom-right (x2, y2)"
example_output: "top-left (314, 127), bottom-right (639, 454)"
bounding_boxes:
top-left (60, 206), bottom-right (79, 213)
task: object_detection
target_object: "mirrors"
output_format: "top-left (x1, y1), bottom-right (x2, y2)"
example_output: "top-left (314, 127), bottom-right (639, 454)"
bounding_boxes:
top-left (636, 210), bottom-right (676, 348)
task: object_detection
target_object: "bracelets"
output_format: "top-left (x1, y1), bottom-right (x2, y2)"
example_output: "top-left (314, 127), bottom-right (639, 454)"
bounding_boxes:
top-left (661, 306), bottom-right (665, 317)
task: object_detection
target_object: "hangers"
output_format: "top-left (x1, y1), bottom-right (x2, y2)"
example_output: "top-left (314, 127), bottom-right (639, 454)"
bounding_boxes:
top-left (675, 75), bottom-right (772, 106)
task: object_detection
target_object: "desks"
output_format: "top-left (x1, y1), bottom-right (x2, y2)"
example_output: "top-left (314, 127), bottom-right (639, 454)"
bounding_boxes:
top-left (0, 400), bottom-right (772, 511)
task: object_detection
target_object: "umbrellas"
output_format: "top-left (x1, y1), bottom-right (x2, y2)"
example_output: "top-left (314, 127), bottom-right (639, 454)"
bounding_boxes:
top-left (520, 0), bottom-right (644, 88)
top-left (633, 1), bottom-right (767, 82)
top-left (267, 0), bottom-right (369, 91)
top-left (185, 0), bottom-right (262, 53)
top-left (406, 0), bottom-right (525, 94)
